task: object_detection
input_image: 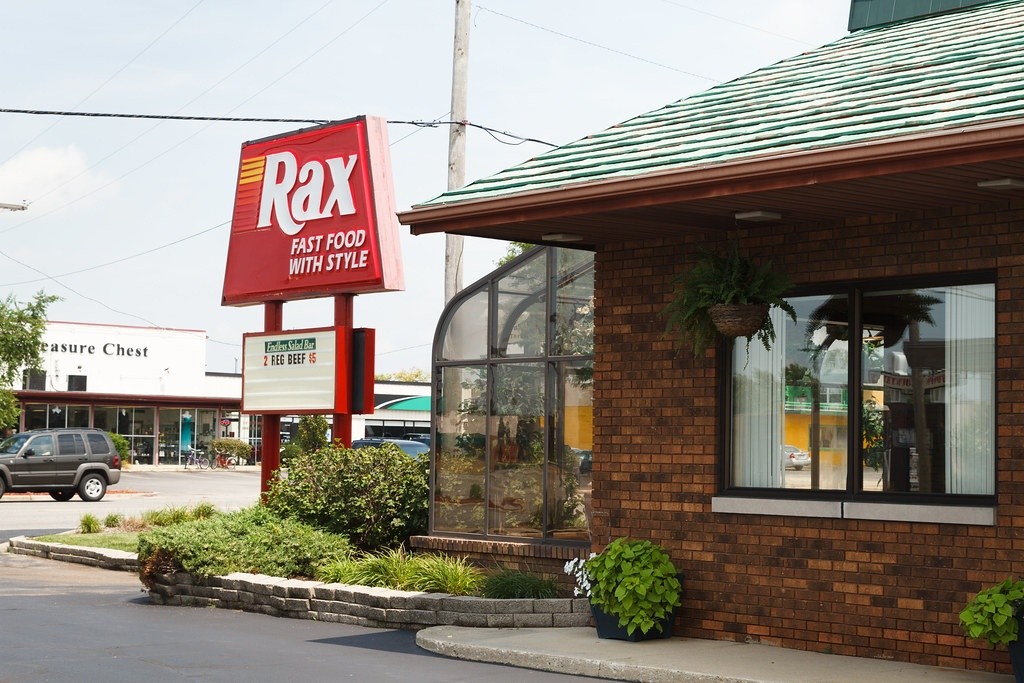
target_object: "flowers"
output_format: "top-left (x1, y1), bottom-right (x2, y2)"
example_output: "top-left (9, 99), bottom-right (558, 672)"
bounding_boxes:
top-left (562, 536), bottom-right (684, 636)
top-left (538, 296), bottom-right (595, 389)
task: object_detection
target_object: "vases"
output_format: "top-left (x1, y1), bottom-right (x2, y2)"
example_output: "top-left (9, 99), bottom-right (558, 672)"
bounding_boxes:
top-left (588, 596), bottom-right (678, 643)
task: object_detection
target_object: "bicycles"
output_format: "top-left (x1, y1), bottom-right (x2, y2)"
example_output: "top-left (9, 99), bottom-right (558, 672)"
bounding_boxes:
top-left (210, 446), bottom-right (237, 470)
top-left (184, 444), bottom-right (210, 471)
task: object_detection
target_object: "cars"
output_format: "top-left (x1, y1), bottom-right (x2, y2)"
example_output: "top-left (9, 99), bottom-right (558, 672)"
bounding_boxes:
top-left (780, 444), bottom-right (811, 471)
top-left (570, 447), bottom-right (592, 475)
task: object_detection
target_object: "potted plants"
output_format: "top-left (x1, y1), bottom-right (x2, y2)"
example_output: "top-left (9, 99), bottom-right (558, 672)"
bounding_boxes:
top-left (958, 576), bottom-right (1024, 683)
top-left (804, 289), bottom-right (942, 364)
top-left (655, 238), bottom-right (797, 371)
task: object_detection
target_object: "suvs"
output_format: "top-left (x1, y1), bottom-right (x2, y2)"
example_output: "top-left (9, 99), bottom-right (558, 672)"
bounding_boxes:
top-left (0, 426), bottom-right (122, 502)
top-left (401, 432), bottom-right (431, 446)
top-left (352, 436), bottom-right (430, 462)
